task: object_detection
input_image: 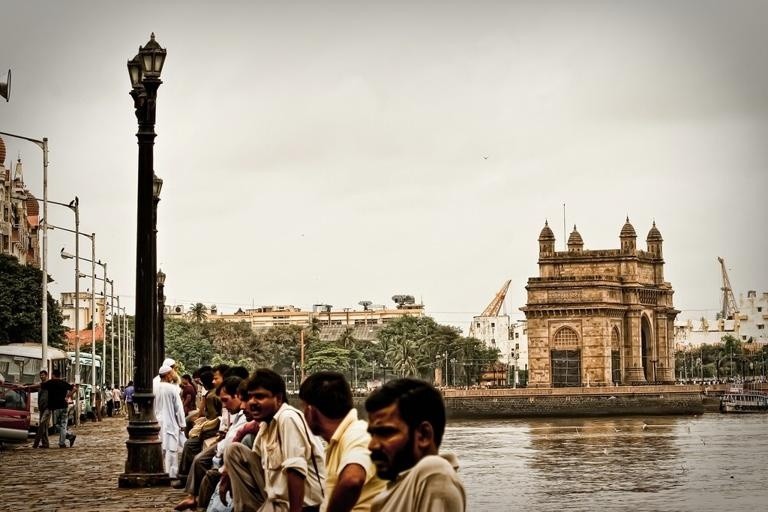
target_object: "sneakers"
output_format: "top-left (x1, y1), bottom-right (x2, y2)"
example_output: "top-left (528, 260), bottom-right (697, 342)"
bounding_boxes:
top-left (60, 435), bottom-right (76, 447)
top-left (32, 444), bottom-right (49, 448)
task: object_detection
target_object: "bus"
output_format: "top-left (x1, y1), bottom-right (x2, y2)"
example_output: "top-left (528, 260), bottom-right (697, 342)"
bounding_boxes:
top-left (0, 341), bottom-right (105, 435)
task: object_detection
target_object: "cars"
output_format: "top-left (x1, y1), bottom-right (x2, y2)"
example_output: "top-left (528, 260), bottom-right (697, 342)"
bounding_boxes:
top-left (0, 383), bottom-right (30, 446)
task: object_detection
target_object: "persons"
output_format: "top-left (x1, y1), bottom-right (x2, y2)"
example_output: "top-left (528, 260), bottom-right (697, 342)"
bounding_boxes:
top-left (13, 370), bottom-right (52, 448)
top-left (19, 369), bottom-right (77, 448)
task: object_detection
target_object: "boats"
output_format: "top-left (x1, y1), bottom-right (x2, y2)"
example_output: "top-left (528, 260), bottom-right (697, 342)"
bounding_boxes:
top-left (720, 394), bottom-right (768, 414)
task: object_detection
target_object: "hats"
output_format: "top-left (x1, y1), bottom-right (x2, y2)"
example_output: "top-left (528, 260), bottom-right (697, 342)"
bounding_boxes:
top-left (159, 358), bottom-right (176, 374)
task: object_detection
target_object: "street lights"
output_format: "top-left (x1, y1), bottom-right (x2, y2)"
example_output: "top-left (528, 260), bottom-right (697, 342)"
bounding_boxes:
top-left (118, 31), bottom-right (168, 490)
top-left (10, 189), bottom-right (134, 427)
